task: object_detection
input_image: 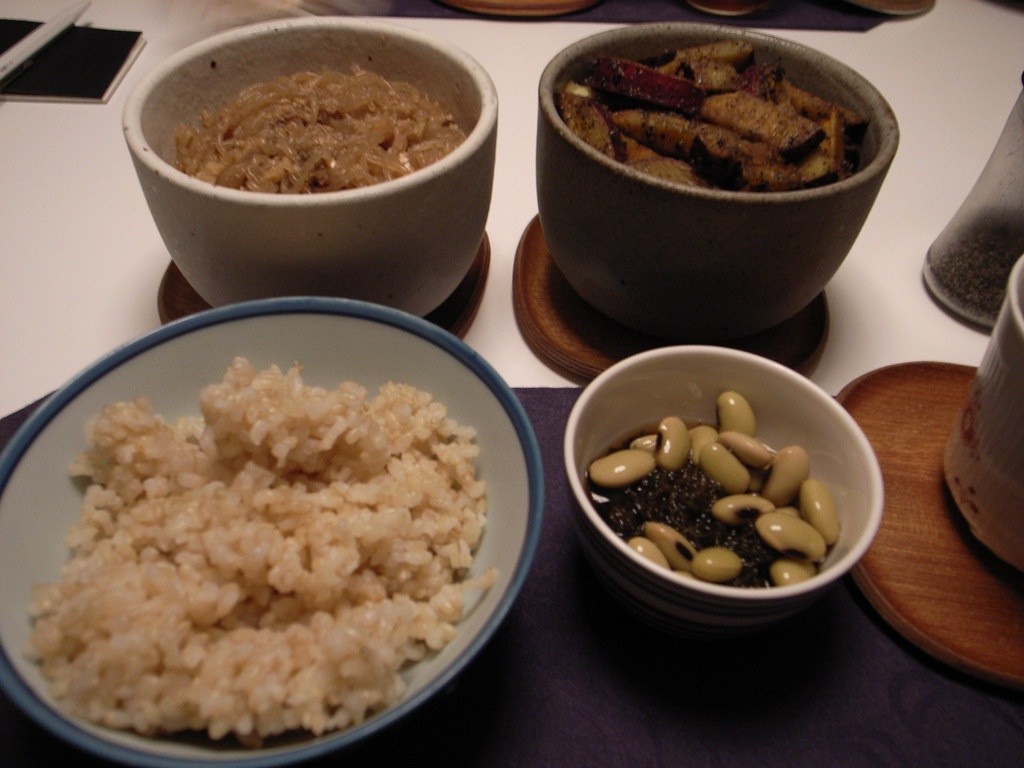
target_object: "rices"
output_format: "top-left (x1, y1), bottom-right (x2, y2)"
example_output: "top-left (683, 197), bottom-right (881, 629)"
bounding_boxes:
top-left (25, 354), bottom-right (498, 744)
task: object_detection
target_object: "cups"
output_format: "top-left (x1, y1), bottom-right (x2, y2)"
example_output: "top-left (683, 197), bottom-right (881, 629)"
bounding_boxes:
top-left (565, 344), bottom-right (885, 633)
top-left (122, 18), bottom-right (500, 318)
top-left (943, 252), bottom-right (1023, 568)
top-left (537, 23), bottom-right (899, 343)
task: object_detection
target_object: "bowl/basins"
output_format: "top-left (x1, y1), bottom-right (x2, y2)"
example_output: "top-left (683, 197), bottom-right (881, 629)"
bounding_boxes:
top-left (0, 295), bottom-right (545, 768)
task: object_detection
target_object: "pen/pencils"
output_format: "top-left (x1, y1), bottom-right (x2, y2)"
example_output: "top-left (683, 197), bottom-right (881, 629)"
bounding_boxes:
top-left (0, 0), bottom-right (93, 89)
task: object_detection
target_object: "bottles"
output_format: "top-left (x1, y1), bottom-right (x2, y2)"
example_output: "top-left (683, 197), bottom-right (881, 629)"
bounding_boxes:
top-left (922, 72), bottom-right (1024, 328)
